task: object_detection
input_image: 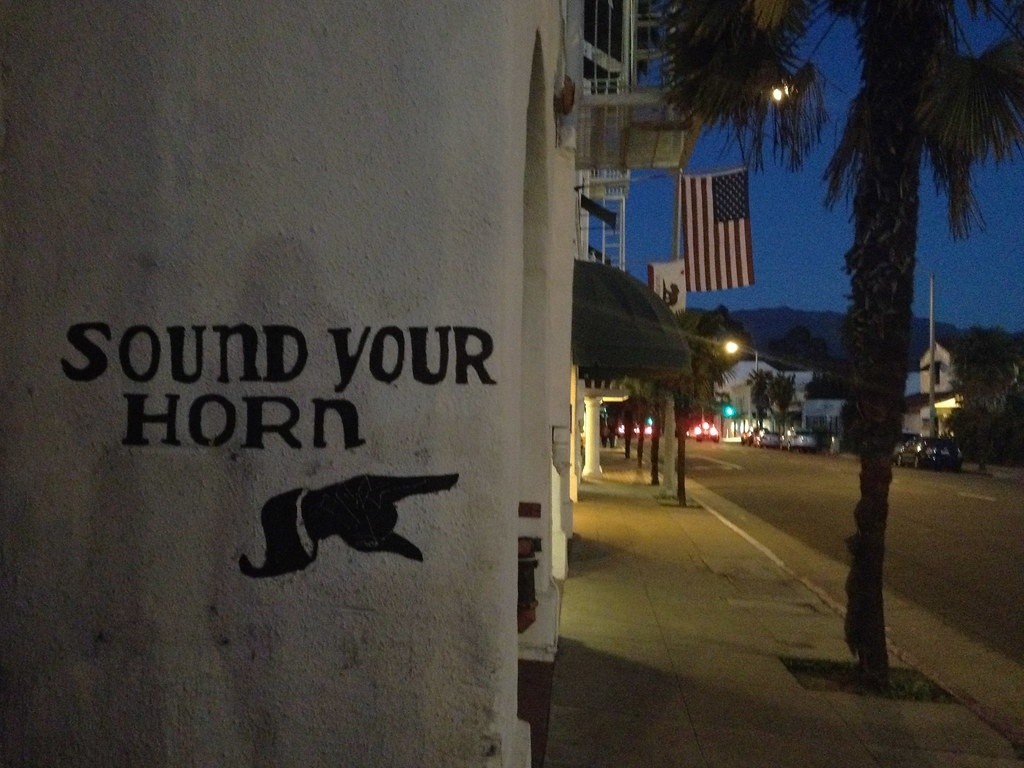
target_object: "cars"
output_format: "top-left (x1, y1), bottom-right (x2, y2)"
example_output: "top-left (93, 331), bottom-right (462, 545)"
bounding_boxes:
top-left (893, 438), bottom-right (962, 471)
top-left (740, 427), bottom-right (818, 453)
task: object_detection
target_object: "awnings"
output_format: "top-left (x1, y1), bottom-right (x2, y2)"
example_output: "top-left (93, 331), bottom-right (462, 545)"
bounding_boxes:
top-left (572, 258), bottom-right (695, 398)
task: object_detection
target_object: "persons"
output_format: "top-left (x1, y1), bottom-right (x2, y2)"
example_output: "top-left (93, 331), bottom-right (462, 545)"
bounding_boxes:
top-left (601, 423), bottom-right (615, 447)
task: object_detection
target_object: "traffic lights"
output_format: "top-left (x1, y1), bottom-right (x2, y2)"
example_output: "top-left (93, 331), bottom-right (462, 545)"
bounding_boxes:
top-left (727, 408), bottom-right (733, 415)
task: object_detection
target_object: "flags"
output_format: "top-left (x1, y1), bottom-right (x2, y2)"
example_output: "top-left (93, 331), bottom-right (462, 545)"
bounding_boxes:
top-left (683, 169), bottom-right (755, 292)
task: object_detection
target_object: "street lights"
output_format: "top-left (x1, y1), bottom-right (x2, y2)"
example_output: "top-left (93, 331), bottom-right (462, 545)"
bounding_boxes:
top-left (726, 342), bottom-right (758, 426)
